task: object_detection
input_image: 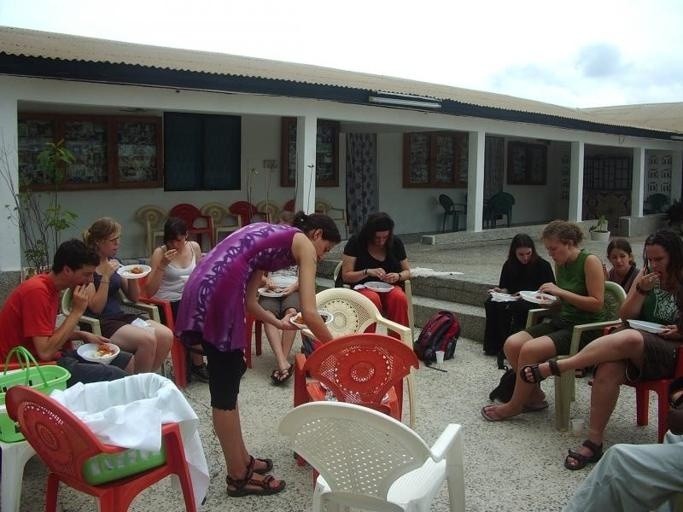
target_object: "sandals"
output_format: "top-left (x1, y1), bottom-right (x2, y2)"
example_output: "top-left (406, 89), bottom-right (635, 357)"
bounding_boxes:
top-left (520, 358), bottom-right (563, 385)
top-left (564, 438), bottom-right (605, 471)
top-left (225, 473), bottom-right (286, 498)
top-left (248, 455), bottom-right (275, 475)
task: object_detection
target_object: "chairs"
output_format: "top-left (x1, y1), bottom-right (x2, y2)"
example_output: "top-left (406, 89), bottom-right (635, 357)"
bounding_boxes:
top-left (294, 334), bottom-right (419, 488)
top-left (587, 324), bottom-right (683, 445)
top-left (439, 193), bottom-right (516, 233)
top-left (279, 399), bottom-right (464, 512)
top-left (527, 279), bottom-right (627, 430)
top-left (0, 193), bottom-right (413, 394)
top-left (5, 384), bottom-right (196, 512)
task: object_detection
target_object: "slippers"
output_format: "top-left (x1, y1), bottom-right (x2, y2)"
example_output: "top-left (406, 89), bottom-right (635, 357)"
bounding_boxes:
top-left (270, 362), bottom-right (296, 386)
top-left (481, 399), bottom-right (549, 423)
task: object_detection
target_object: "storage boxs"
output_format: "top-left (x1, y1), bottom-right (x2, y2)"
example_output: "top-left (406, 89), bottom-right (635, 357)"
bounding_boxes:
top-left (50, 373), bottom-right (181, 484)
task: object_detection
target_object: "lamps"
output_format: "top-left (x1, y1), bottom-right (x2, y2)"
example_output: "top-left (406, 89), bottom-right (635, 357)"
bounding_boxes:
top-left (246, 167), bottom-right (261, 220)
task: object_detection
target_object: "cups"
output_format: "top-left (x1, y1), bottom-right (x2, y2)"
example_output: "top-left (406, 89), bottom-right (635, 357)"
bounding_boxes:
top-left (435, 350), bottom-right (444, 363)
top-left (571, 418), bottom-right (584, 434)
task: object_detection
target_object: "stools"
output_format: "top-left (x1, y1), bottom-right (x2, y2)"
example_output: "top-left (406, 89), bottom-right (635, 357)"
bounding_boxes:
top-left (1, 438), bottom-right (37, 510)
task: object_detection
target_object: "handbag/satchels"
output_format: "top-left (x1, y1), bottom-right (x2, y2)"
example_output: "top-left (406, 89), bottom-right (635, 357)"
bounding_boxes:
top-left (488, 369), bottom-right (516, 404)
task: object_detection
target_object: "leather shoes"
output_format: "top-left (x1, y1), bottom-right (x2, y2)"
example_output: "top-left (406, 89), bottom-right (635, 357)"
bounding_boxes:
top-left (188, 364), bottom-right (209, 381)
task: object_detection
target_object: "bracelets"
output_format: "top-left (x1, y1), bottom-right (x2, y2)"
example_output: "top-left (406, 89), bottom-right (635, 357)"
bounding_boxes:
top-left (635, 283), bottom-right (652, 296)
top-left (100, 280), bottom-right (110, 283)
top-left (398, 273), bottom-right (401, 281)
top-left (157, 266), bottom-right (165, 272)
top-left (363, 268), bottom-right (367, 277)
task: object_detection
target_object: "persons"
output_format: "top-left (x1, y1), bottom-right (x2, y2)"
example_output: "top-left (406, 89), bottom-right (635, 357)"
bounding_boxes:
top-left (559, 375), bottom-right (683, 512)
top-left (142, 218), bottom-right (209, 383)
top-left (520, 228), bottom-right (683, 470)
top-left (172, 212), bottom-right (342, 497)
top-left (335, 211), bottom-right (411, 342)
top-left (256, 214), bottom-right (305, 387)
top-left (71, 217), bottom-right (175, 376)
top-left (575, 238), bottom-right (642, 386)
top-left (0, 238), bottom-right (135, 383)
top-left (480, 220), bottom-right (608, 422)
top-left (484, 233), bottom-right (557, 356)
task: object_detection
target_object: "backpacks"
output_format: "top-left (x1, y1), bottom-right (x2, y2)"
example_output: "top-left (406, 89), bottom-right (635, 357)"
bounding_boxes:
top-left (415, 309), bottom-right (462, 365)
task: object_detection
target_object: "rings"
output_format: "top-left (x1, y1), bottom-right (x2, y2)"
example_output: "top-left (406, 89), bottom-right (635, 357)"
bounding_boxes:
top-left (393, 278), bottom-right (394, 281)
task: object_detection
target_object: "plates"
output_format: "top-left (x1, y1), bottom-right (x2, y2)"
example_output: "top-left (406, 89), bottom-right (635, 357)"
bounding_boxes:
top-left (626, 317), bottom-right (671, 334)
top-left (365, 281), bottom-right (394, 293)
top-left (520, 290), bottom-right (557, 305)
top-left (257, 287), bottom-right (286, 297)
top-left (115, 264), bottom-right (150, 279)
top-left (491, 292), bottom-right (520, 301)
top-left (75, 342), bottom-right (120, 363)
top-left (289, 310), bottom-right (333, 330)
